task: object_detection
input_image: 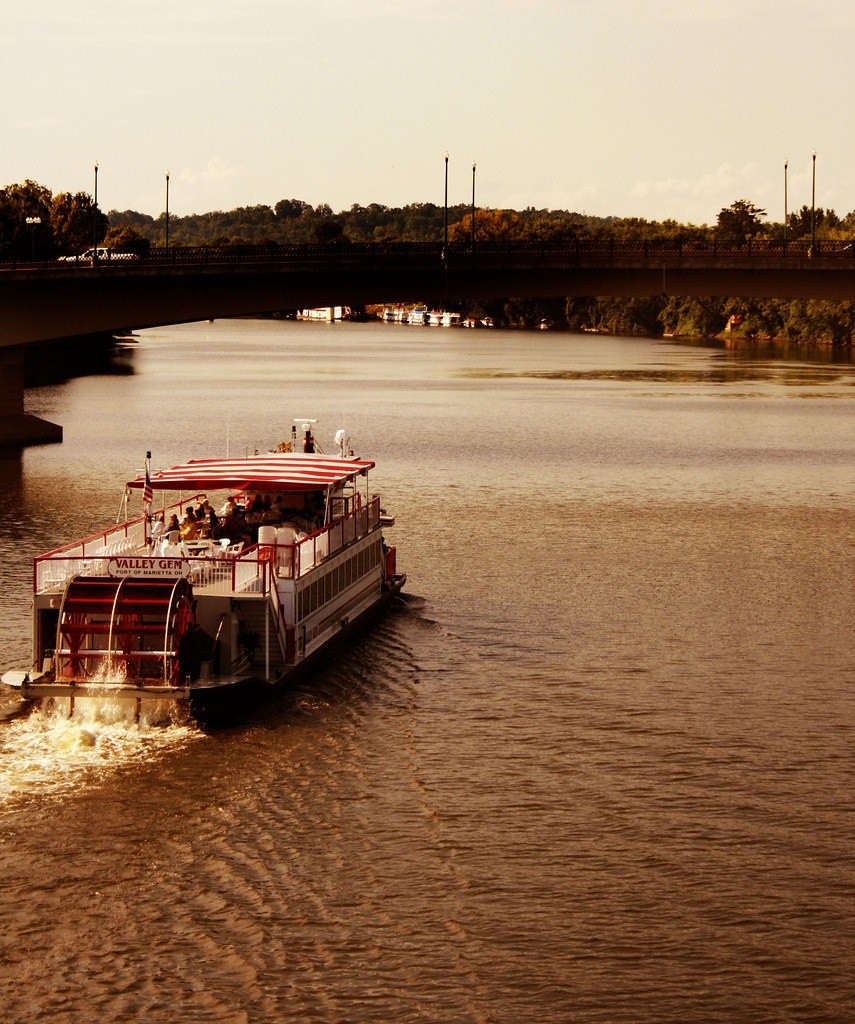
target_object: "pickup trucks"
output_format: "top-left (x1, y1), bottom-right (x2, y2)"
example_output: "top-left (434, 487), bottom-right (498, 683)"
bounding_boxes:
top-left (66, 246), bottom-right (141, 262)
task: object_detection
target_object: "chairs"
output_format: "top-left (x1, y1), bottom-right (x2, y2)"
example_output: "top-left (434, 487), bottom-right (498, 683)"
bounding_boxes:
top-left (181, 538), bottom-right (244, 582)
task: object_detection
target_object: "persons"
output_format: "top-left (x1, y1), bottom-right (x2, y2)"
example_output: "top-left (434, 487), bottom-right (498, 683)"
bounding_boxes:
top-left (223, 496), bottom-right (252, 544)
top-left (248, 491), bottom-right (286, 519)
top-left (147, 500), bottom-right (224, 541)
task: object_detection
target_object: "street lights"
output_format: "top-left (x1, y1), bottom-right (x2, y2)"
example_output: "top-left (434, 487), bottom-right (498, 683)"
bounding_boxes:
top-left (470, 159), bottom-right (478, 243)
top-left (443, 148), bottom-right (450, 245)
top-left (93, 160), bottom-right (99, 245)
top-left (165, 169), bottom-right (170, 247)
top-left (810, 152), bottom-right (817, 238)
top-left (25, 216), bottom-right (41, 262)
top-left (783, 159), bottom-right (790, 239)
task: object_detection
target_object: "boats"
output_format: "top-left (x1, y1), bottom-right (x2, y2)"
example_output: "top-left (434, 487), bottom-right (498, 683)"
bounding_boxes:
top-left (296, 303), bottom-right (556, 330)
top-left (0, 419), bottom-right (409, 732)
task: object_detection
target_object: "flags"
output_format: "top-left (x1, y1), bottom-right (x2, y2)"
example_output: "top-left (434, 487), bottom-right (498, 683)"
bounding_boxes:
top-left (139, 464), bottom-right (156, 519)
